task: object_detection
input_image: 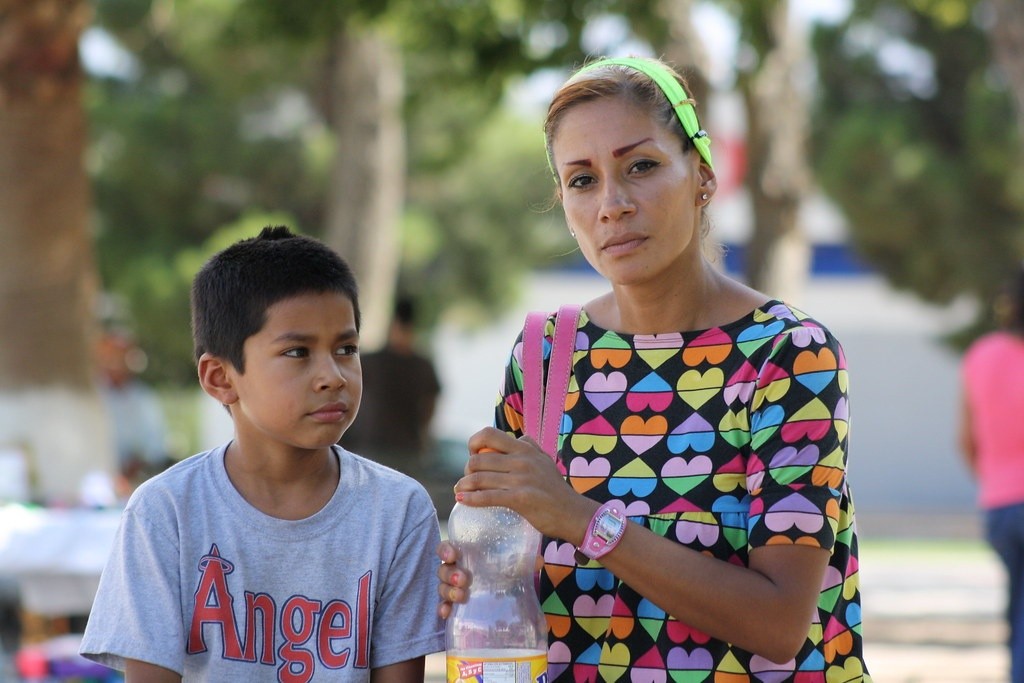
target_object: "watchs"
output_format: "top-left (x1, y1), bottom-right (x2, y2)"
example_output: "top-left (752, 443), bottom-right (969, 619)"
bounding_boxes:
top-left (578, 499), bottom-right (626, 560)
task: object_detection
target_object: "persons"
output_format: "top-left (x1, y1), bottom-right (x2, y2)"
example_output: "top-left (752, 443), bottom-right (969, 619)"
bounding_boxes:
top-left (439, 56), bottom-right (880, 683)
top-left (88, 328), bottom-right (179, 492)
top-left (338, 288), bottom-right (441, 480)
top-left (79, 222), bottom-right (445, 683)
top-left (962, 269), bottom-right (1024, 683)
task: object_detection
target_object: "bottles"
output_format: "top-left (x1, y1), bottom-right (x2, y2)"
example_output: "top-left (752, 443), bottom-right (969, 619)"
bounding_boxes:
top-left (447, 444), bottom-right (549, 682)
